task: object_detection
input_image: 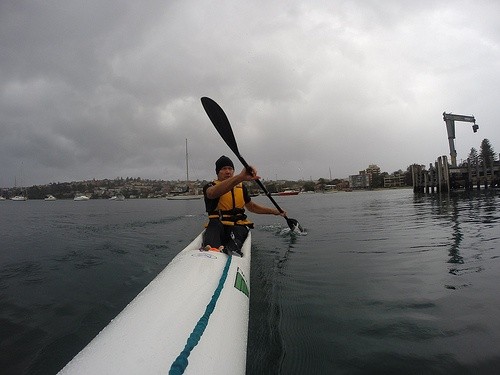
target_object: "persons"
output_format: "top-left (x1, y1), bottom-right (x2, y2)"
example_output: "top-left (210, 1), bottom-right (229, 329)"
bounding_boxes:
top-left (203, 155), bottom-right (288, 257)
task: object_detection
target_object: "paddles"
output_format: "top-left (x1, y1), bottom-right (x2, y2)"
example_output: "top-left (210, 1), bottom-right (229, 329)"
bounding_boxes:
top-left (200, 96), bottom-right (304, 232)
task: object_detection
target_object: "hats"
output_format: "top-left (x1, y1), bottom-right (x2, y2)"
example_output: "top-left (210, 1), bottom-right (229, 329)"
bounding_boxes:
top-left (214, 155), bottom-right (235, 175)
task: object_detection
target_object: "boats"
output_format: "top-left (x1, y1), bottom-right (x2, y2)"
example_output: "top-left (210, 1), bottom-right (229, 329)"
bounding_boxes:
top-left (278, 189), bottom-right (299, 195)
top-left (12, 195), bottom-right (27, 201)
top-left (44, 195), bottom-right (56, 201)
top-left (74, 196), bottom-right (88, 201)
top-left (166, 194), bottom-right (205, 200)
top-left (53, 226), bottom-right (253, 375)
